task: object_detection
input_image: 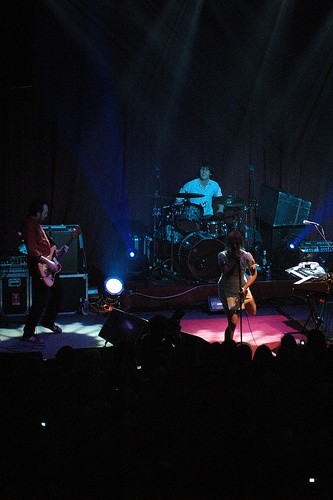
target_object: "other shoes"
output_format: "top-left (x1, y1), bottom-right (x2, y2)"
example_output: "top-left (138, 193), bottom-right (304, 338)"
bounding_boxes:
top-left (23, 335), bottom-right (44, 344)
top-left (40, 322), bottom-right (62, 334)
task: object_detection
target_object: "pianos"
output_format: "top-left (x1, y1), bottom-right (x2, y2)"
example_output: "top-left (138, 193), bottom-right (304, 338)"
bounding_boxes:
top-left (284, 261), bottom-right (333, 342)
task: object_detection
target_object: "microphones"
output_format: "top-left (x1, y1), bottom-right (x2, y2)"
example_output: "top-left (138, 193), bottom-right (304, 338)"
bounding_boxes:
top-left (236, 257), bottom-right (241, 262)
top-left (303, 220), bottom-right (320, 226)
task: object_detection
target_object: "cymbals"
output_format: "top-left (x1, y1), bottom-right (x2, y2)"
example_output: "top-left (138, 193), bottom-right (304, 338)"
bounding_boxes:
top-left (162, 191), bottom-right (206, 199)
top-left (212, 194), bottom-right (250, 207)
top-left (144, 193), bottom-right (173, 200)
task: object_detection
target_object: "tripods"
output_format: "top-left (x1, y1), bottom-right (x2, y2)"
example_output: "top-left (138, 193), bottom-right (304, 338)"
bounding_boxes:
top-left (152, 197), bottom-right (188, 276)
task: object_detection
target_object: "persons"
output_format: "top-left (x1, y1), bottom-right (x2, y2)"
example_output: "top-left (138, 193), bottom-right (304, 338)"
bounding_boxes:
top-left (217, 230), bottom-right (257, 340)
top-left (174, 160), bottom-right (223, 216)
top-left (0, 326), bottom-right (333, 500)
top-left (23, 198), bottom-right (70, 343)
top-left (279, 240), bottom-right (329, 280)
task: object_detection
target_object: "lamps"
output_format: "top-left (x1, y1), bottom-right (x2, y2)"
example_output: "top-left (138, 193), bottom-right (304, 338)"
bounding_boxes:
top-left (103, 276), bottom-right (125, 297)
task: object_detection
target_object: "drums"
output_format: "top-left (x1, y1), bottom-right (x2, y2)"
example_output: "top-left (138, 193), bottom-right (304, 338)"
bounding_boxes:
top-left (173, 202), bottom-right (205, 233)
top-left (177, 229), bottom-right (227, 283)
top-left (202, 219), bottom-right (228, 238)
top-left (156, 204), bottom-right (177, 223)
top-left (147, 217), bottom-right (186, 266)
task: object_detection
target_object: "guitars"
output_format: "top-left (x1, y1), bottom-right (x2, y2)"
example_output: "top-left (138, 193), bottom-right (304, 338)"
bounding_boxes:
top-left (36, 227), bottom-right (81, 287)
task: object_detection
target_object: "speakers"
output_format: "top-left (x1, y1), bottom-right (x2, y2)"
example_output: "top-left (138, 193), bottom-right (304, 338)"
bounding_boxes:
top-left (99, 310), bottom-right (149, 352)
top-left (44, 228), bottom-right (83, 274)
top-left (255, 184), bottom-right (312, 268)
top-left (205, 296), bottom-right (224, 313)
